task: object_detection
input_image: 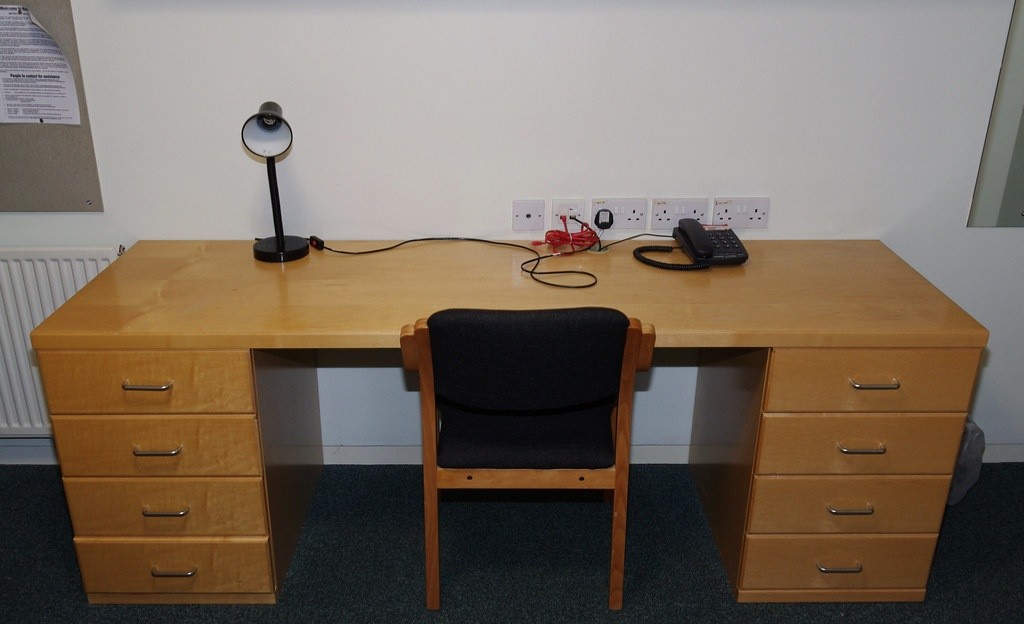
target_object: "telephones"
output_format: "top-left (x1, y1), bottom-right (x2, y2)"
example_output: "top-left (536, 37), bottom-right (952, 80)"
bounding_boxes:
top-left (633, 217), bottom-right (750, 272)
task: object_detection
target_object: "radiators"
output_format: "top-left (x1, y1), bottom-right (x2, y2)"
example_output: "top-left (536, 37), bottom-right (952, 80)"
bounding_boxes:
top-left (0, 249), bottom-right (116, 439)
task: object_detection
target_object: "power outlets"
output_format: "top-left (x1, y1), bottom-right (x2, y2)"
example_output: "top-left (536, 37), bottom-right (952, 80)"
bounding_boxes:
top-left (512, 197), bottom-right (770, 230)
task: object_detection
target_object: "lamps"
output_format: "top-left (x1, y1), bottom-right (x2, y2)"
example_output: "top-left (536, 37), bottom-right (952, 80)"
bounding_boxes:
top-left (240, 100), bottom-right (310, 263)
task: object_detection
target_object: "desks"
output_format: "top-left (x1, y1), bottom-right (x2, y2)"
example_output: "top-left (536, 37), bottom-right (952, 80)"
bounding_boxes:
top-left (30, 239), bottom-right (991, 605)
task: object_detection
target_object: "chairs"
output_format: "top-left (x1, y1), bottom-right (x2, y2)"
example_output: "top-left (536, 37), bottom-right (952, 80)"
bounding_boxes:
top-left (400, 306), bottom-right (656, 610)
